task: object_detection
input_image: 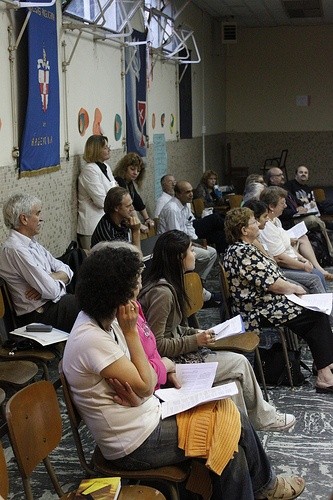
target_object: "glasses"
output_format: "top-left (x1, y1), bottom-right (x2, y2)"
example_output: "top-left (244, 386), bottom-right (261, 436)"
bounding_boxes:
top-left (246, 218), bottom-right (260, 225)
top-left (271, 174), bottom-right (286, 177)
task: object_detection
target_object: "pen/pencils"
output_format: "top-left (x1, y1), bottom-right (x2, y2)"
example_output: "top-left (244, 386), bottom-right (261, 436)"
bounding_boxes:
top-left (198, 330), bottom-right (219, 336)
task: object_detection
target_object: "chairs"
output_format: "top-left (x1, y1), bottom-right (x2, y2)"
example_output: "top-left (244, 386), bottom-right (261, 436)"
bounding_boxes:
top-left (0, 183), bottom-right (333, 500)
top-left (259, 149), bottom-right (290, 184)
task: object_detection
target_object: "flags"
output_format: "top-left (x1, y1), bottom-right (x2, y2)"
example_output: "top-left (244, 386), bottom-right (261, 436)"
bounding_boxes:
top-left (122, 27), bottom-right (147, 157)
top-left (18, 2), bottom-right (63, 179)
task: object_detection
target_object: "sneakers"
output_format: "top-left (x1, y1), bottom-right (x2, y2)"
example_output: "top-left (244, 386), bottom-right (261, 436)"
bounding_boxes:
top-left (259, 412), bottom-right (296, 433)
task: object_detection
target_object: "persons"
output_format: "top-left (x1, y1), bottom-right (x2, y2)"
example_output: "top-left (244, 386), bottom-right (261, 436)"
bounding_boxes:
top-left (63, 246), bottom-right (306, 500)
top-left (77, 136), bottom-right (119, 285)
top-left (134, 229), bottom-right (296, 430)
top-left (92, 185), bottom-right (146, 272)
top-left (223, 208), bottom-right (333, 395)
top-left (87, 240), bottom-right (180, 398)
top-left (239, 200), bottom-right (333, 332)
top-left (112, 152), bottom-right (156, 235)
top-left (157, 164), bottom-right (333, 293)
top-left (1, 194), bottom-right (80, 332)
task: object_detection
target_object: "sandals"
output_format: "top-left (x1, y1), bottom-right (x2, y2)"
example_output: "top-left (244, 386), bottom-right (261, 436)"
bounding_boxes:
top-left (257, 474), bottom-right (305, 500)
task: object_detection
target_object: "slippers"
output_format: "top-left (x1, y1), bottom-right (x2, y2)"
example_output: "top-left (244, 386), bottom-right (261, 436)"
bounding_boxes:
top-left (313, 383), bottom-right (333, 393)
